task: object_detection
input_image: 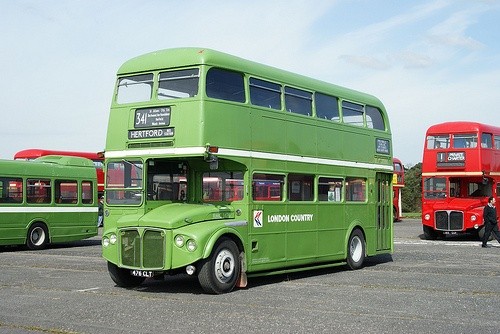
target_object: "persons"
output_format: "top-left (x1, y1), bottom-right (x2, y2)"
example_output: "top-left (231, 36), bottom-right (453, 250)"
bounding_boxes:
top-left (98, 196), bottom-right (103, 227)
top-left (436, 141), bottom-right (443, 149)
top-left (482, 197), bottom-right (500, 247)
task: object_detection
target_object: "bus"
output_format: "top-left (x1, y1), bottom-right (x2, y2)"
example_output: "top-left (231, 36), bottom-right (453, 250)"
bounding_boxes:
top-left (94, 47), bottom-right (394, 295)
top-left (178, 158), bottom-right (405, 224)
top-left (9, 148), bottom-right (136, 203)
top-left (0, 156), bottom-right (98, 250)
top-left (416, 122), bottom-right (500, 241)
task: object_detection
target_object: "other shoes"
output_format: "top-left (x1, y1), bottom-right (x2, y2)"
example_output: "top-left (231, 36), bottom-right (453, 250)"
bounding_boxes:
top-left (482, 245), bottom-right (491, 248)
